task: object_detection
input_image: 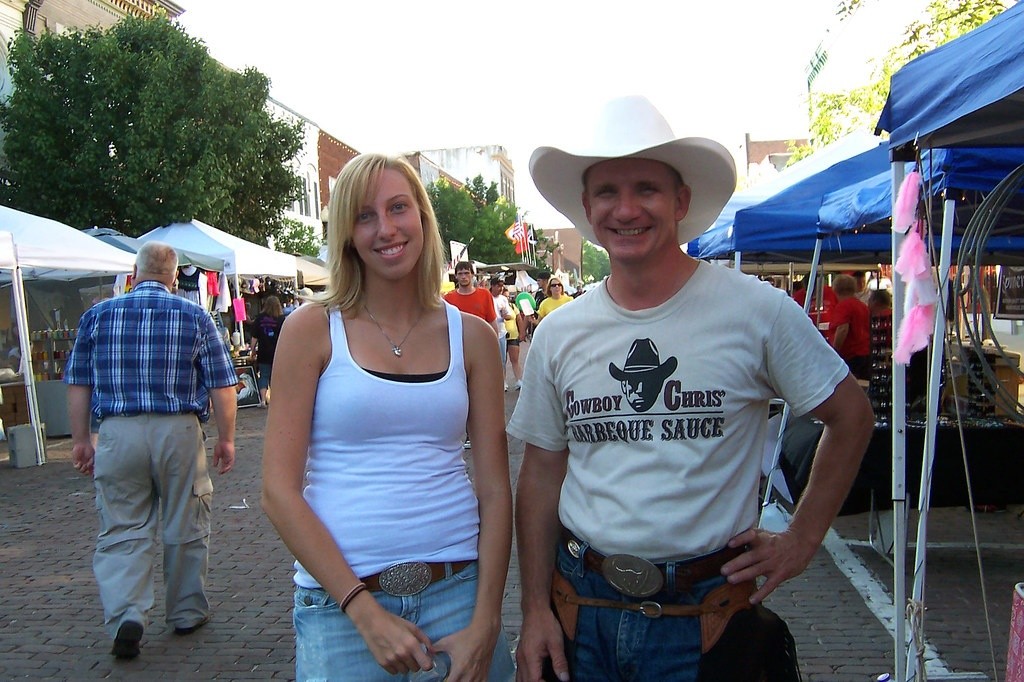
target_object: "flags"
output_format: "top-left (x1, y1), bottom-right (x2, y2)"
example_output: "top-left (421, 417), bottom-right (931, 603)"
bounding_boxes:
top-left (505, 214), bottom-right (537, 254)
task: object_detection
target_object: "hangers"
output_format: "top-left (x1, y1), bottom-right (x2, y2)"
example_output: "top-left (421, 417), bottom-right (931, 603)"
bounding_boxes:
top-left (273, 278), bottom-right (294, 296)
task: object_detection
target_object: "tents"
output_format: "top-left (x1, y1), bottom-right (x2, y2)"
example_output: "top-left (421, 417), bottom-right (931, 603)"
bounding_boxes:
top-left (688, 0), bottom-right (1024, 682)
top-left (0, 204), bottom-right (329, 465)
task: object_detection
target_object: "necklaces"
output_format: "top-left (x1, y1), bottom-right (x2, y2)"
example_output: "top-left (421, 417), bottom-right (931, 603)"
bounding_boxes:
top-left (364, 306), bottom-right (424, 357)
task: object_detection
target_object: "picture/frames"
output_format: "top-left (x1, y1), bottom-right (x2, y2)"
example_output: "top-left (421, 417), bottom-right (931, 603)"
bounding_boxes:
top-left (234, 364), bottom-right (263, 408)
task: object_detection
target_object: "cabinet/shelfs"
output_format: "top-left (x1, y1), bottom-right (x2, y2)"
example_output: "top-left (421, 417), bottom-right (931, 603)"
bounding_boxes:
top-left (28, 337), bottom-right (75, 437)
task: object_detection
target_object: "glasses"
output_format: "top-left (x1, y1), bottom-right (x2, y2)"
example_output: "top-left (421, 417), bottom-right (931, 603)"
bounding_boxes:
top-left (550, 283), bottom-right (562, 287)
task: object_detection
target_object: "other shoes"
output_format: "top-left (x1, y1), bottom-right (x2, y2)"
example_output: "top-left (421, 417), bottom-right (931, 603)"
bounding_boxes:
top-left (504, 381), bottom-right (509, 391)
top-left (258, 402), bottom-right (266, 408)
top-left (513, 381), bottom-right (523, 391)
top-left (175, 616), bottom-right (210, 635)
top-left (111, 620), bottom-right (143, 659)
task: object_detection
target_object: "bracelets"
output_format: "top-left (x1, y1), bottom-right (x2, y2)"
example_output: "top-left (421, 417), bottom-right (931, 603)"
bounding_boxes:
top-left (340, 582), bottom-right (367, 613)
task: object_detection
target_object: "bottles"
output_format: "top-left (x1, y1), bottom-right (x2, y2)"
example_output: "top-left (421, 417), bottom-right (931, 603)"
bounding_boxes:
top-left (31, 328), bottom-right (78, 381)
top-left (407, 641), bottom-right (449, 682)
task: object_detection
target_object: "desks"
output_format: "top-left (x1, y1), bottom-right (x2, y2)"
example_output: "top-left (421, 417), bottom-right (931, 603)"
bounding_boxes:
top-left (780, 408), bottom-right (1024, 516)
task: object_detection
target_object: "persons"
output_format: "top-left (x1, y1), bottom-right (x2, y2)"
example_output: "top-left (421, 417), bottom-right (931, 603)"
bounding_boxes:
top-left (250, 295), bottom-right (285, 407)
top-left (868, 271), bottom-right (891, 291)
top-left (259, 153), bottom-right (513, 682)
top-left (851, 271), bottom-right (874, 306)
top-left (529, 271), bottom-right (574, 327)
top-left (794, 271), bottom-right (834, 312)
top-left (444, 261), bottom-right (499, 335)
top-left (489, 279), bottom-right (525, 392)
top-left (870, 289), bottom-right (892, 362)
top-left (828, 273), bottom-right (871, 380)
top-left (62, 240), bottom-right (239, 657)
top-left (505, 97), bottom-right (876, 682)
top-left (300, 287), bottom-right (313, 305)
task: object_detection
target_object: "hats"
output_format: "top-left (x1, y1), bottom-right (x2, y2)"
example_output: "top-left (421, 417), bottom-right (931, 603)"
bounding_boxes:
top-left (527, 95), bottom-right (737, 251)
top-left (300, 288), bottom-right (313, 299)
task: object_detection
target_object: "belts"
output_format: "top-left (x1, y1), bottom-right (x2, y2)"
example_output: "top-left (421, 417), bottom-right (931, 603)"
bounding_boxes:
top-left (558, 521), bottom-right (750, 599)
top-left (360, 556), bottom-right (473, 596)
